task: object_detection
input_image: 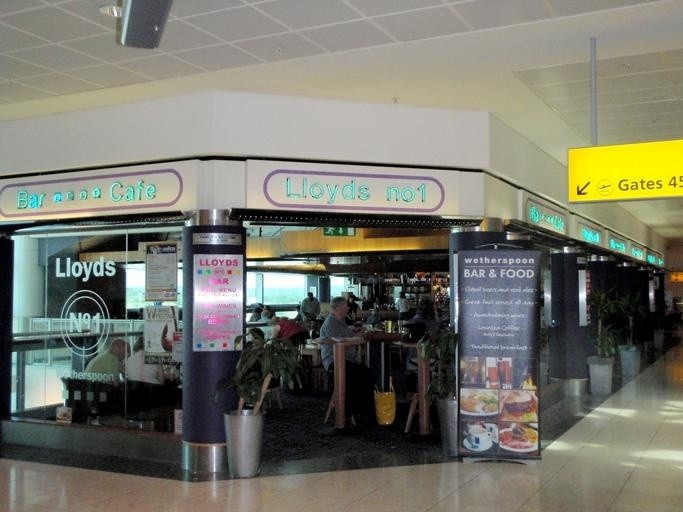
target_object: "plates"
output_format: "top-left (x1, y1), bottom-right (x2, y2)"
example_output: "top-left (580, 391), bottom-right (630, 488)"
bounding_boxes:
top-left (460, 389), bottom-right (498, 418)
top-left (499, 427), bottom-right (538, 453)
top-left (462, 435), bottom-right (493, 452)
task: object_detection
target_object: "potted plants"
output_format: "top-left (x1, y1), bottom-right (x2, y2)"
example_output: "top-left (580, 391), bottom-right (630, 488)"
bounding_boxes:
top-left (423, 330), bottom-right (459, 458)
top-left (587, 283), bottom-right (623, 396)
top-left (213, 329), bottom-right (302, 479)
top-left (652, 304), bottom-right (668, 349)
top-left (616, 290), bottom-right (647, 377)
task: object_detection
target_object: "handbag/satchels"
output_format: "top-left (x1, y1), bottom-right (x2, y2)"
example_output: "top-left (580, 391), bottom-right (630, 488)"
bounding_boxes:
top-left (374, 376), bottom-right (396, 426)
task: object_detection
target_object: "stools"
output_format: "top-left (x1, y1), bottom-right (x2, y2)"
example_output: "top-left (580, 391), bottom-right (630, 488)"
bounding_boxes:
top-left (266, 384), bottom-right (283, 411)
top-left (324, 373), bottom-right (357, 426)
top-left (404, 392), bottom-right (433, 433)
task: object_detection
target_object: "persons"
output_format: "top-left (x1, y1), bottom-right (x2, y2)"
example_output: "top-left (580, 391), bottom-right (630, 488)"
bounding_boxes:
top-left (84, 338), bottom-right (131, 427)
top-left (318, 295), bottom-right (376, 434)
top-left (124, 336), bottom-right (175, 432)
top-left (247, 287), bottom-right (443, 394)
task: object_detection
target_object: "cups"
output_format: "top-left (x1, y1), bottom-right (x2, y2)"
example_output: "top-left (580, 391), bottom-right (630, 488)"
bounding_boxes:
top-left (488, 366), bottom-right (499, 388)
top-left (498, 364), bottom-right (514, 390)
top-left (383, 321), bottom-right (393, 334)
top-left (465, 358), bottom-right (481, 385)
top-left (467, 427), bottom-right (492, 448)
top-left (397, 320), bottom-right (407, 335)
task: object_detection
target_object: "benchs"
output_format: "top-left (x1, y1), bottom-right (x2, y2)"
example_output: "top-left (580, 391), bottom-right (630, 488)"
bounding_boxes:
top-left (62, 375), bottom-right (183, 429)
